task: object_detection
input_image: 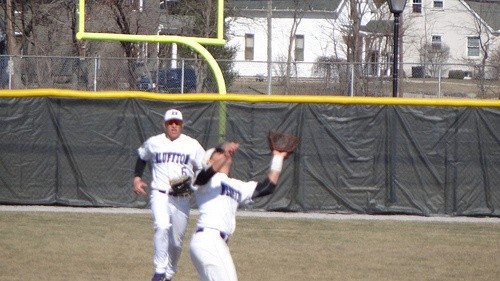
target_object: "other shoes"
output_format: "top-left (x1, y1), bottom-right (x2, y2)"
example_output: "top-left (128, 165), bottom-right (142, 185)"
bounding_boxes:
top-left (165, 278), bottom-right (171, 281)
top-left (152, 272), bottom-right (166, 281)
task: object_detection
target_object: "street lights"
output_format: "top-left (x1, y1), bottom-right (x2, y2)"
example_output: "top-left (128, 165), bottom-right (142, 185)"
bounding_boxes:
top-left (387, 0), bottom-right (408, 99)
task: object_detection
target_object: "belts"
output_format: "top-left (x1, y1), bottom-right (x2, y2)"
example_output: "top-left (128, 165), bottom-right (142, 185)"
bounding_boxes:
top-left (159, 190), bottom-right (190, 196)
top-left (196, 227), bottom-right (228, 243)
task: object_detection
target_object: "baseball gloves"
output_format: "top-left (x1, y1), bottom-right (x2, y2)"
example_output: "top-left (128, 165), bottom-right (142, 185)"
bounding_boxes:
top-left (169, 175), bottom-right (192, 197)
top-left (268, 131), bottom-right (301, 159)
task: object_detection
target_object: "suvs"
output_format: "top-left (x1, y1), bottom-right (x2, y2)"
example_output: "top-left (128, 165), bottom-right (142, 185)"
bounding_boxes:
top-left (139, 68), bottom-right (197, 93)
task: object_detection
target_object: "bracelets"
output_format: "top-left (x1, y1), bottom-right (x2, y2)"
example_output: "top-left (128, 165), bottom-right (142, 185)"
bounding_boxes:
top-left (271, 155), bottom-right (283, 172)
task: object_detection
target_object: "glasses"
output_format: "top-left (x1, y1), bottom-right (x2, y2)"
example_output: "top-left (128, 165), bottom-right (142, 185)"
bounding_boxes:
top-left (165, 121), bottom-right (182, 126)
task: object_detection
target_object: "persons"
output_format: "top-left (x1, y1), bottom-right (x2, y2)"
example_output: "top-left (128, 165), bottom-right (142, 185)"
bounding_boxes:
top-left (134, 109), bottom-right (206, 281)
top-left (188, 129), bottom-right (300, 281)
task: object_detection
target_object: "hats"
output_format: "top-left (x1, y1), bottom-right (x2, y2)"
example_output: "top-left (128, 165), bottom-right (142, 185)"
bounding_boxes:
top-left (164, 109), bottom-right (183, 122)
top-left (202, 148), bottom-right (224, 171)
